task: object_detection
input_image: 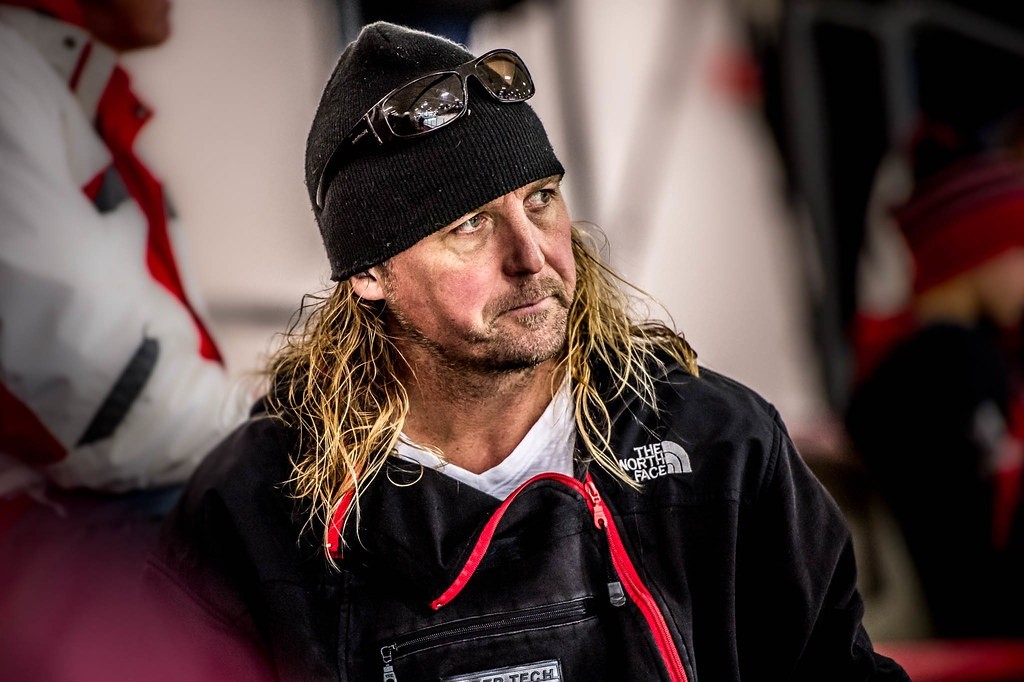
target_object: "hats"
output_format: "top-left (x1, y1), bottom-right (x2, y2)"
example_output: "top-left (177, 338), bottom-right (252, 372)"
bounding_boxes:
top-left (304, 20), bottom-right (566, 282)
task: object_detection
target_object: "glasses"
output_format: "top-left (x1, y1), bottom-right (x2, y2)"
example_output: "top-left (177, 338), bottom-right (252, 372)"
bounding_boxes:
top-left (315, 49), bottom-right (536, 210)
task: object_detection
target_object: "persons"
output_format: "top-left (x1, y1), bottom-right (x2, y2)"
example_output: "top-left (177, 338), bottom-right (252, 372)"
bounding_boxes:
top-left (184, 18), bottom-right (911, 682)
top-left (0, 0), bottom-right (255, 493)
